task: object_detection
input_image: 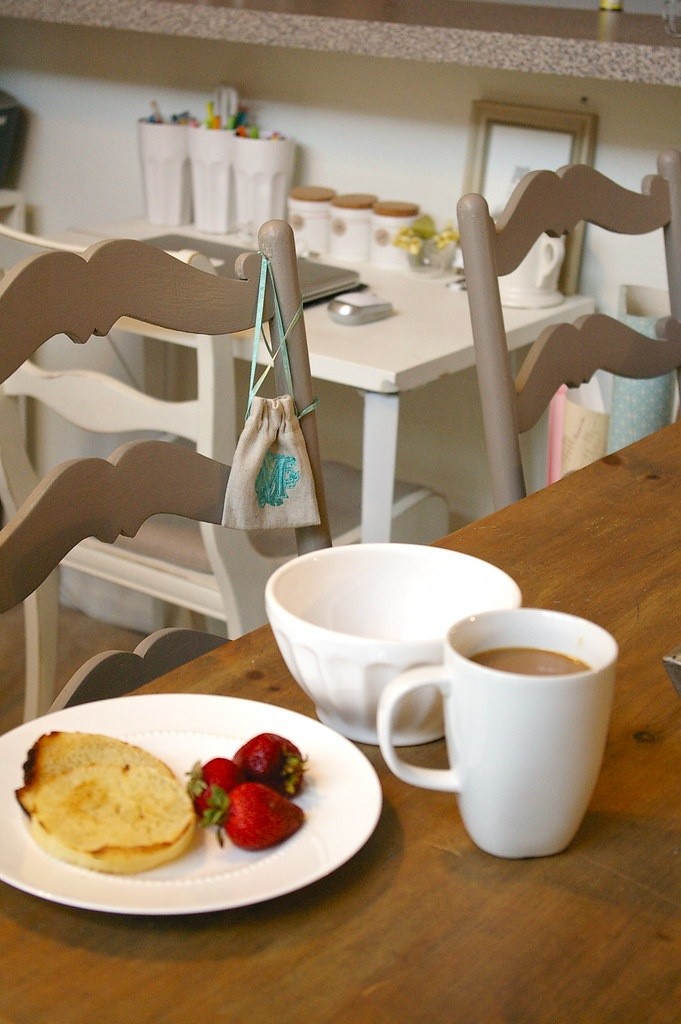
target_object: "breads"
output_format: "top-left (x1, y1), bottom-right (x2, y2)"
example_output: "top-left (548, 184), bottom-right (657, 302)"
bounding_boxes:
top-left (16, 730), bottom-right (197, 873)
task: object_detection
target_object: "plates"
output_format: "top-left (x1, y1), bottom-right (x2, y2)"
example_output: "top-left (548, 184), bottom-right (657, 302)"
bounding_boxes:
top-left (0, 691), bottom-right (387, 916)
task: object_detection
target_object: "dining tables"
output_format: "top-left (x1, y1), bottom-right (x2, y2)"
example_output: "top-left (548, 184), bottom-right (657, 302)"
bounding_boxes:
top-left (0, 412), bottom-right (679, 1024)
top-left (59, 230), bottom-right (558, 636)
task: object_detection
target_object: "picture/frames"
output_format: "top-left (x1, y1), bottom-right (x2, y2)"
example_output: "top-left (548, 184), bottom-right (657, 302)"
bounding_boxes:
top-left (445, 90), bottom-right (605, 290)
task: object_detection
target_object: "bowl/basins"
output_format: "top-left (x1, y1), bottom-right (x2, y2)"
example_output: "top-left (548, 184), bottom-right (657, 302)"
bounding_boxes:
top-left (264, 542), bottom-right (526, 750)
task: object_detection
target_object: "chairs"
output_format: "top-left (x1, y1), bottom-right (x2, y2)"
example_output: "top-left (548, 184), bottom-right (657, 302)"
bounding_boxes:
top-left (1, 223), bottom-right (456, 719)
top-left (1, 210), bottom-right (335, 741)
top-left (457, 156), bottom-right (681, 499)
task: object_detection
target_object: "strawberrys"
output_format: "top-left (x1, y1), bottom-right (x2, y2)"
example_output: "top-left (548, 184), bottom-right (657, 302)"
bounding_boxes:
top-left (185, 732), bottom-right (309, 850)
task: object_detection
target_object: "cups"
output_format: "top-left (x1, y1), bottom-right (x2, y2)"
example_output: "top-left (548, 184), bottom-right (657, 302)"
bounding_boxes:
top-left (232, 128), bottom-right (299, 235)
top-left (288, 187), bottom-right (335, 253)
top-left (186, 123), bottom-right (244, 235)
top-left (376, 605), bottom-right (623, 861)
top-left (138, 115), bottom-right (197, 227)
top-left (329, 192), bottom-right (379, 259)
top-left (495, 227), bottom-right (568, 308)
top-left (373, 200), bottom-right (421, 273)
